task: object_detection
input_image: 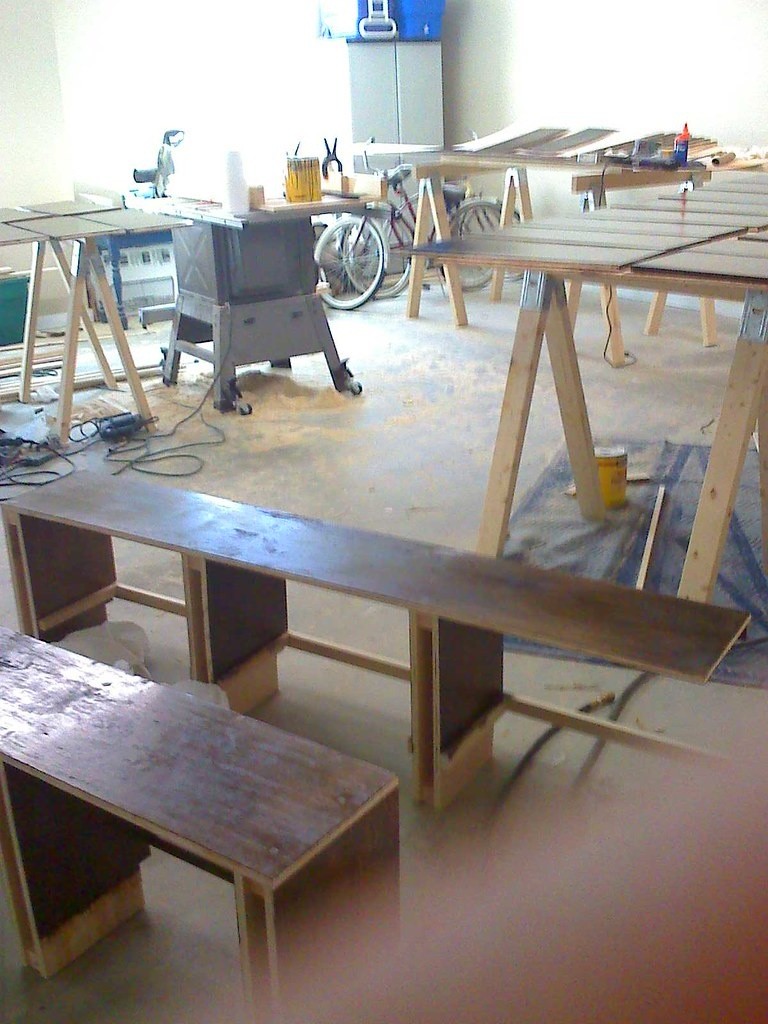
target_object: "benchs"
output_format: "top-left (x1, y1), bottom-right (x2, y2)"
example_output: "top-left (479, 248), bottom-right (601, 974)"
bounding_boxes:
top-left (0, 630), bottom-right (404, 1016)
top-left (1, 469), bottom-right (755, 807)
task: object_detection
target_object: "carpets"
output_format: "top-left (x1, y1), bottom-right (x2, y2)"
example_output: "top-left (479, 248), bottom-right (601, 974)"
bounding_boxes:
top-left (492, 434), bottom-right (768, 695)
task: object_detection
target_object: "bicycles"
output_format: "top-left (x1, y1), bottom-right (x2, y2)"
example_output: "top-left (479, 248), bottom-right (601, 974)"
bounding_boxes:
top-left (308, 166), bottom-right (532, 310)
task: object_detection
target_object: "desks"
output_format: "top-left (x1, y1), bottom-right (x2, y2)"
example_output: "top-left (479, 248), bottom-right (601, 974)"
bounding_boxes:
top-left (0, 200), bottom-right (192, 440)
top-left (395, 120), bottom-right (767, 363)
top-left (396, 164), bottom-right (767, 603)
